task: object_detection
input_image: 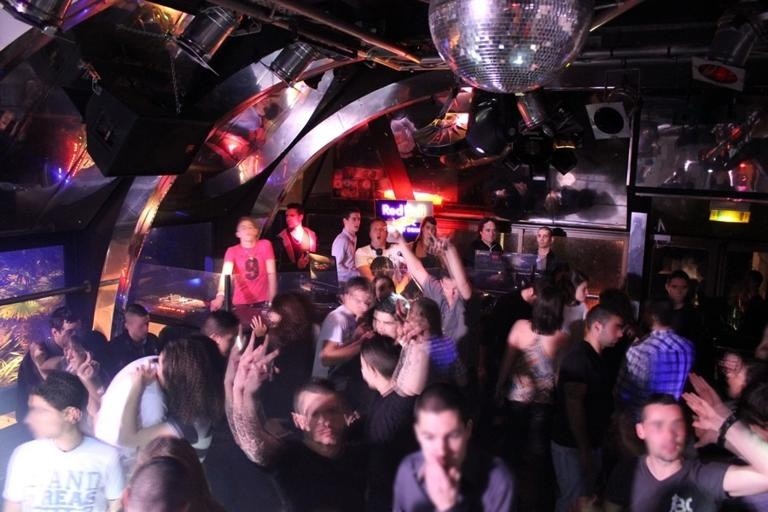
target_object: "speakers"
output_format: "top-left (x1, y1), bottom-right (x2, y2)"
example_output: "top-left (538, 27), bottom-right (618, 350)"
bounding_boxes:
top-left (84, 89), bottom-right (215, 176)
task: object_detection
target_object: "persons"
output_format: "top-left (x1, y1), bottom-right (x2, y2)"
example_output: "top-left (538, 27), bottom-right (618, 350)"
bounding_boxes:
top-left (0, 203), bottom-right (768, 512)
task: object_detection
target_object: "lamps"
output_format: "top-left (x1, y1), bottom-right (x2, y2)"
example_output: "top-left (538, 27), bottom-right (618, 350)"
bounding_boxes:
top-left (170, 4), bottom-right (242, 76)
top-left (586, 61), bottom-right (634, 141)
top-left (0, 0), bottom-right (78, 45)
top-left (257, 36), bottom-right (319, 95)
top-left (516, 91), bottom-right (549, 130)
top-left (691, 1), bottom-right (768, 92)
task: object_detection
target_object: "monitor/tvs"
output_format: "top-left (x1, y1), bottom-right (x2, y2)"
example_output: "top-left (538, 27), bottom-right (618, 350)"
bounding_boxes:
top-left (376, 200), bottom-right (432, 244)
